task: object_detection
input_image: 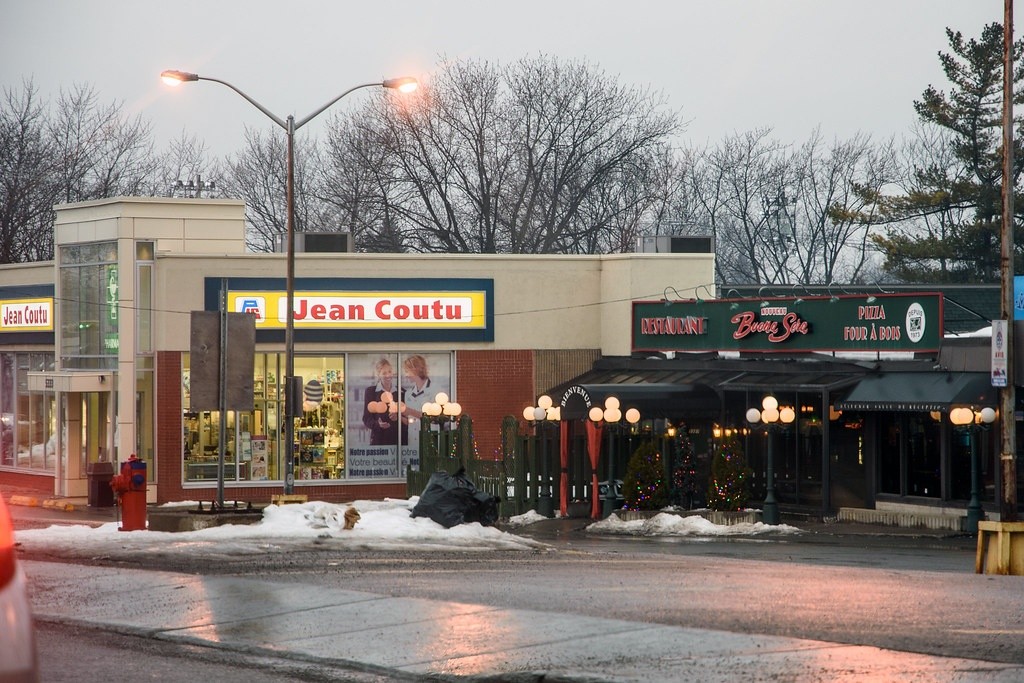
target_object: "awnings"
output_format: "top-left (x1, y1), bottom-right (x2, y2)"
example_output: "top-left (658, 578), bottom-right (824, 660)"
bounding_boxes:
top-left (833, 371), bottom-right (997, 413)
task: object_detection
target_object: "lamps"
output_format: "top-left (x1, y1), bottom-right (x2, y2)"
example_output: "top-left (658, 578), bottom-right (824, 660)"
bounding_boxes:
top-left (98, 376), bottom-right (105, 384)
top-left (758, 286), bottom-right (787, 309)
top-left (828, 282), bottom-right (853, 306)
top-left (792, 284), bottom-right (824, 306)
top-left (695, 285), bottom-right (719, 306)
top-left (727, 289), bottom-right (755, 311)
top-left (663, 286), bottom-right (685, 308)
top-left (860, 274), bottom-right (894, 304)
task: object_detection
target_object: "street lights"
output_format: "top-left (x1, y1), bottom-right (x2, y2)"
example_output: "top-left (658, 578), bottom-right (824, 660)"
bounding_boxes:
top-left (949, 407), bottom-right (996, 532)
top-left (524, 394), bottom-right (561, 518)
top-left (159, 68), bottom-right (418, 495)
top-left (746, 395), bottom-right (796, 525)
top-left (421, 390), bottom-right (463, 470)
top-left (589, 395), bottom-right (641, 520)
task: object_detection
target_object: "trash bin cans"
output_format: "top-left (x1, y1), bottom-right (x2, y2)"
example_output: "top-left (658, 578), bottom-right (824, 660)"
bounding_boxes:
top-left (86, 462), bottom-right (114, 507)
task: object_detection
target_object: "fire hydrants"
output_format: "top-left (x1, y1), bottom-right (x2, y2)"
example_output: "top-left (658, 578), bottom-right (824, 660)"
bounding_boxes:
top-left (110, 453), bottom-right (147, 532)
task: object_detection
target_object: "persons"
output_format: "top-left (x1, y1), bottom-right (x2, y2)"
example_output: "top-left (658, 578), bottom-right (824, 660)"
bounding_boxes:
top-left (362, 358), bottom-right (408, 446)
top-left (388, 355), bottom-right (437, 445)
